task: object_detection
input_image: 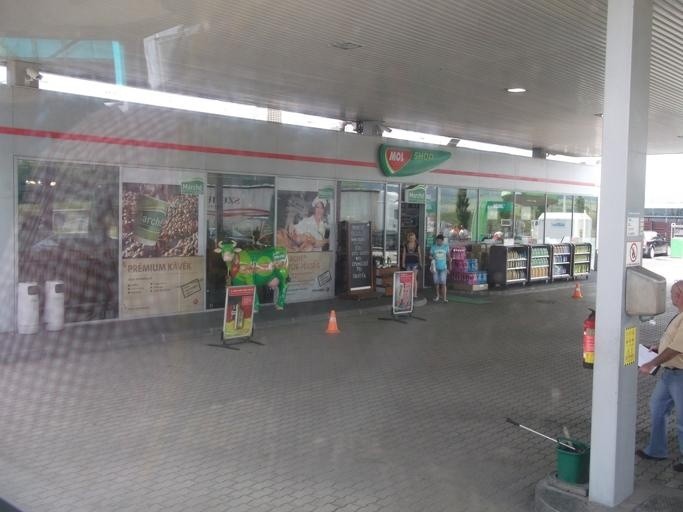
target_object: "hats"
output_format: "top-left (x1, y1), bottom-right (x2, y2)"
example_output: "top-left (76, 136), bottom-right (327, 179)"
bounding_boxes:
top-left (312, 195), bottom-right (328, 209)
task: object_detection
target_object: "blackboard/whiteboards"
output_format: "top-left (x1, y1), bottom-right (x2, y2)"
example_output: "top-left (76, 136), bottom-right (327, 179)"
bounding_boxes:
top-left (348, 220), bottom-right (377, 295)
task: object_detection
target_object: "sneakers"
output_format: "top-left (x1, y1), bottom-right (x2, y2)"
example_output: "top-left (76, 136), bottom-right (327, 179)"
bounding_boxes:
top-left (672, 461), bottom-right (683, 472)
top-left (635, 446), bottom-right (670, 460)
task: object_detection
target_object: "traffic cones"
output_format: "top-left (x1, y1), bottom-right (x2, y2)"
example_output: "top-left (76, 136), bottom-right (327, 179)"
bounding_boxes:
top-left (571, 282), bottom-right (582, 298)
top-left (325, 310), bottom-right (341, 334)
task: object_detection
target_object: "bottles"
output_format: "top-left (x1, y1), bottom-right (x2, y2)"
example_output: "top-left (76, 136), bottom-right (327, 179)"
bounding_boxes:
top-left (234, 304), bottom-right (244, 330)
top-left (552, 246), bottom-right (569, 274)
top-left (531, 248), bottom-right (548, 277)
top-left (451, 248), bottom-right (464, 260)
top-left (507, 250), bottom-right (524, 280)
top-left (387, 258), bottom-right (391, 268)
top-left (231, 304), bottom-right (235, 319)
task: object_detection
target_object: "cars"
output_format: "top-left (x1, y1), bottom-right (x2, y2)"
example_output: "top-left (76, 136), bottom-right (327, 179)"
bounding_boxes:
top-left (643, 230), bottom-right (670, 258)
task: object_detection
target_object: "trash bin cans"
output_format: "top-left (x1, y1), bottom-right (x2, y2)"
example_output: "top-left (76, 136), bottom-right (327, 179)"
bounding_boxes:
top-left (17, 281), bottom-right (40, 336)
top-left (670, 237), bottom-right (682, 258)
top-left (43, 280), bottom-right (65, 332)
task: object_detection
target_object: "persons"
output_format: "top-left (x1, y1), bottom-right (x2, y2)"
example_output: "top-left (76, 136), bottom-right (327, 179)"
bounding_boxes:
top-left (402, 232), bottom-right (422, 297)
top-left (483, 231), bottom-right (503, 244)
top-left (429, 234), bottom-right (452, 302)
top-left (395, 288), bottom-right (411, 312)
top-left (443, 223), bottom-right (469, 240)
top-left (292, 196), bottom-right (329, 251)
top-left (639, 280), bottom-right (683, 472)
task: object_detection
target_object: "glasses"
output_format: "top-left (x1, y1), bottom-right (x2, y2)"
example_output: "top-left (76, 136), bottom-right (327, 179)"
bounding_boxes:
top-left (673, 279), bottom-right (683, 294)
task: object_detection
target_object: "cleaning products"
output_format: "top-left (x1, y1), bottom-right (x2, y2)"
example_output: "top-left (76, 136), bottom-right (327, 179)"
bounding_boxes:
top-left (448, 245), bottom-right (487, 285)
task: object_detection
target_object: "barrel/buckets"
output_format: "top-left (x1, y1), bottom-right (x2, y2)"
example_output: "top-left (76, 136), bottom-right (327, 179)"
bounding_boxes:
top-left (554, 435), bottom-right (591, 485)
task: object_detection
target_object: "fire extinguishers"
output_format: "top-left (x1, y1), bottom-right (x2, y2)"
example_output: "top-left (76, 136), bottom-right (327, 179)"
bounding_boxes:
top-left (583, 307), bottom-right (600, 369)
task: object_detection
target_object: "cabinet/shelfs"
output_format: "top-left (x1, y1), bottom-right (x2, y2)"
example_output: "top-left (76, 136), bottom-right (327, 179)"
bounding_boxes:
top-left (375, 243), bottom-right (593, 299)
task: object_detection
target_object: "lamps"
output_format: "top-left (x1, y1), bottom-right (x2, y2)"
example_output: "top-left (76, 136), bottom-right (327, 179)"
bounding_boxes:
top-left (34, 70), bottom-right (362, 135)
top-left (372, 124), bottom-right (548, 160)
